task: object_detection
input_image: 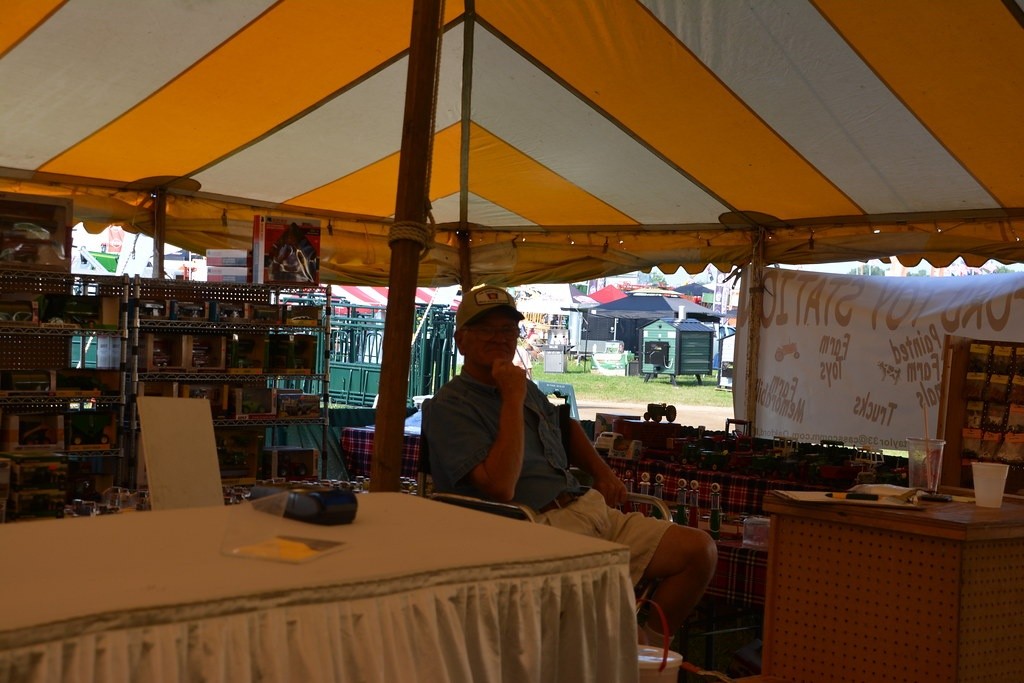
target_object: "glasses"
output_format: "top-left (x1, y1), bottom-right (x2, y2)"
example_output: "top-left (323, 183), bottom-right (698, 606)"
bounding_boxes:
top-left (462, 326), bottom-right (520, 341)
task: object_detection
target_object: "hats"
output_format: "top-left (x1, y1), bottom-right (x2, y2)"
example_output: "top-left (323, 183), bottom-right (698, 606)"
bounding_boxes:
top-left (455, 285), bottom-right (525, 332)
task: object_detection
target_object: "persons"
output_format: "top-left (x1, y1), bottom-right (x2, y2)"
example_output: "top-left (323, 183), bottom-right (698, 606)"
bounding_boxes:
top-left (424, 286), bottom-right (717, 646)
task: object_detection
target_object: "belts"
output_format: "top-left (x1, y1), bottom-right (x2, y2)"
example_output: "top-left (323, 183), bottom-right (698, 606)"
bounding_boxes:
top-left (536, 489), bottom-right (574, 514)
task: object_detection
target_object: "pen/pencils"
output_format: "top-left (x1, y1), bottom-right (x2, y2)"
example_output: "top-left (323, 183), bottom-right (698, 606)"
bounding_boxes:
top-left (825, 492), bottom-right (878, 500)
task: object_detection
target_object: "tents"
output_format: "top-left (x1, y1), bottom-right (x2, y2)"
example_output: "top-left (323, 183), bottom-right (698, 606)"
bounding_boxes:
top-left (162, 249), bottom-right (737, 370)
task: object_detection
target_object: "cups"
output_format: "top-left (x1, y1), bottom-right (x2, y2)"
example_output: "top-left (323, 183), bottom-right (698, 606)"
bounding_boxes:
top-left (906, 437), bottom-right (946, 492)
top-left (972, 462), bottom-right (1009, 508)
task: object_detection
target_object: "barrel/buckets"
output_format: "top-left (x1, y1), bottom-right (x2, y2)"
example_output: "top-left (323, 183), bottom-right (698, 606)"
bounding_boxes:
top-left (636, 598), bottom-right (683, 683)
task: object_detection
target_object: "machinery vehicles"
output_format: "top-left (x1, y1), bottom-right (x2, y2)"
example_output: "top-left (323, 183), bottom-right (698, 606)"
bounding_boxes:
top-left (590, 413), bottom-right (885, 490)
